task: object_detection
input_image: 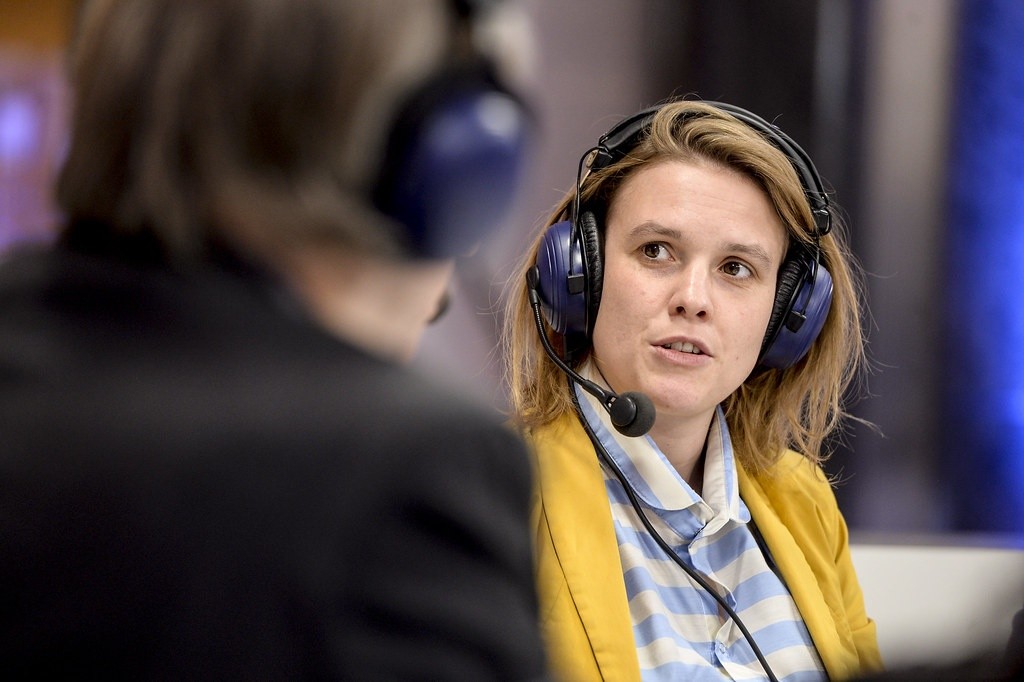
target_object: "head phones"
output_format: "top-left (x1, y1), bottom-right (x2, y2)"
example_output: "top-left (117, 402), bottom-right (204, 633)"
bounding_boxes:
top-left (371, 0), bottom-right (530, 262)
top-left (535, 98), bottom-right (834, 377)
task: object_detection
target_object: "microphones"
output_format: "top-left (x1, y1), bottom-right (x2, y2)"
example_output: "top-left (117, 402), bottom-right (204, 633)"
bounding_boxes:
top-left (526, 265), bottom-right (655, 438)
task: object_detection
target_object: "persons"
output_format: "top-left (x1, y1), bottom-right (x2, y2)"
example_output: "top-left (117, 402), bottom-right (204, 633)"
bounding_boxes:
top-left (495, 100), bottom-right (885, 682)
top-left (0, 2), bottom-right (547, 682)
